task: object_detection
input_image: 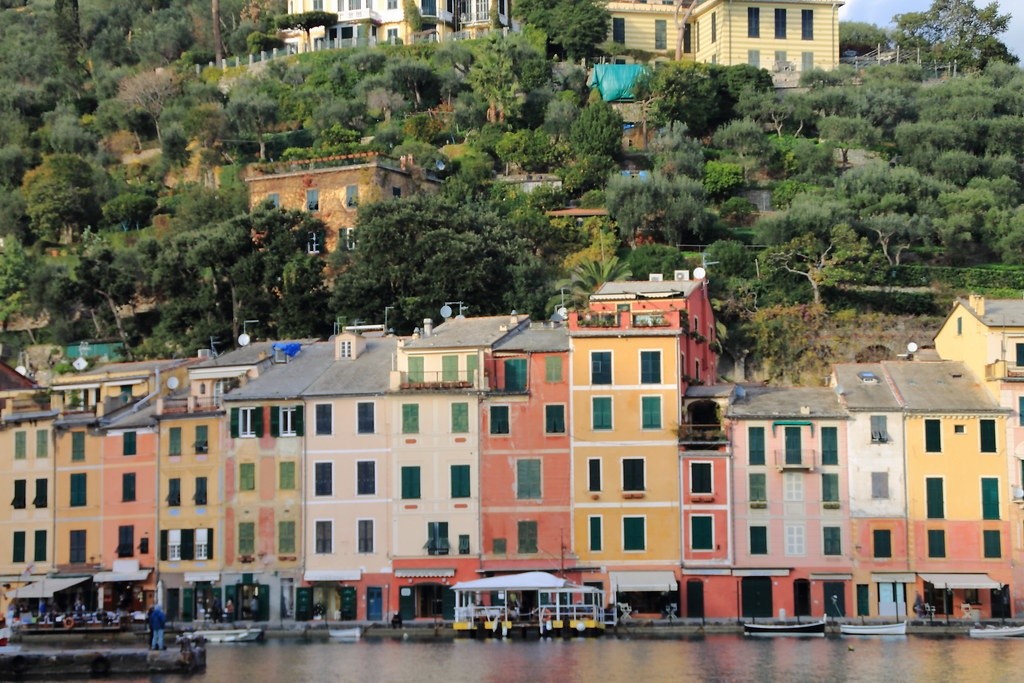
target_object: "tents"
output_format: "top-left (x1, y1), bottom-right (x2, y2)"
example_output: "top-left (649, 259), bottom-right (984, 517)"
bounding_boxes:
top-left (449, 573), bottom-right (567, 621)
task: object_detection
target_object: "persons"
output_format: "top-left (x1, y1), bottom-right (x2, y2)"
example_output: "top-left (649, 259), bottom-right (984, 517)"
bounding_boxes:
top-left (146, 605), bottom-right (169, 651)
top-left (205, 593), bottom-right (260, 625)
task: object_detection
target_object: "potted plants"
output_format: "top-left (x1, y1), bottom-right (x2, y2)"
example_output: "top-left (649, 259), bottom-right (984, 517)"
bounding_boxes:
top-left (680, 311), bottom-right (721, 354)
top-left (670, 424), bottom-right (725, 450)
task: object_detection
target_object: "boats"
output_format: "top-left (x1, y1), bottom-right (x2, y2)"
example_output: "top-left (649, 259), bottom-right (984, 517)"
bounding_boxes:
top-left (744, 612), bottom-right (829, 639)
top-left (305, 623), bottom-right (366, 644)
top-left (838, 618), bottom-right (910, 635)
top-left (182, 625), bottom-right (264, 641)
top-left (969, 624), bottom-right (1024, 638)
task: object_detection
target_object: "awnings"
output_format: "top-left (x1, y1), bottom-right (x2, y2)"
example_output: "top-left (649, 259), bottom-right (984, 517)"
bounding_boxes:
top-left (6, 575), bottom-right (93, 598)
top-left (917, 569), bottom-right (1004, 590)
top-left (96, 569), bottom-right (150, 582)
top-left (609, 570), bottom-right (679, 593)
top-left (395, 569), bottom-right (456, 578)
top-left (304, 571), bottom-right (361, 580)
